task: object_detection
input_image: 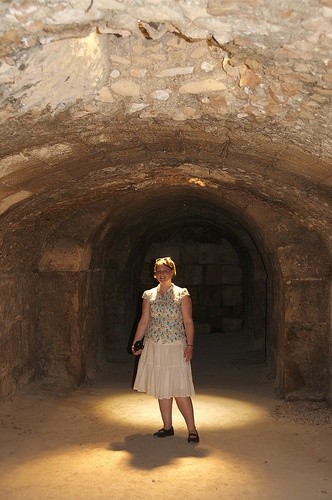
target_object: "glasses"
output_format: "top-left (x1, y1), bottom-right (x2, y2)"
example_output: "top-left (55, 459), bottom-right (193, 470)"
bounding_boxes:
top-left (156, 257), bottom-right (170, 263)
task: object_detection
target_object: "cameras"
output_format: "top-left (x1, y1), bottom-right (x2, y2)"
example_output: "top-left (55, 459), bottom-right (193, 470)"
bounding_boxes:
top-left (134, 341), bottom-right (143, 352)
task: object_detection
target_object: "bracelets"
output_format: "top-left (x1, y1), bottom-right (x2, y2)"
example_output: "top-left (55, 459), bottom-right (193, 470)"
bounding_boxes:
top-left (132, 345), bottom-right (134, 348)
top-left (187, 344), bottom-right (193, 348)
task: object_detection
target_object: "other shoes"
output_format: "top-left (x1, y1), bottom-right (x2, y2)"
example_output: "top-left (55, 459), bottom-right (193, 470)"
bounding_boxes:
top-left (153, 426), bottom-right (174, 437)
top-left (188, 430), bottom-right (199, 443)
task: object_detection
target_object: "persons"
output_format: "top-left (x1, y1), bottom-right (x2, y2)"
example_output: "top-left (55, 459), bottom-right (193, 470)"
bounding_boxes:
top-left (132, 257), bottom-right (200, 445)
